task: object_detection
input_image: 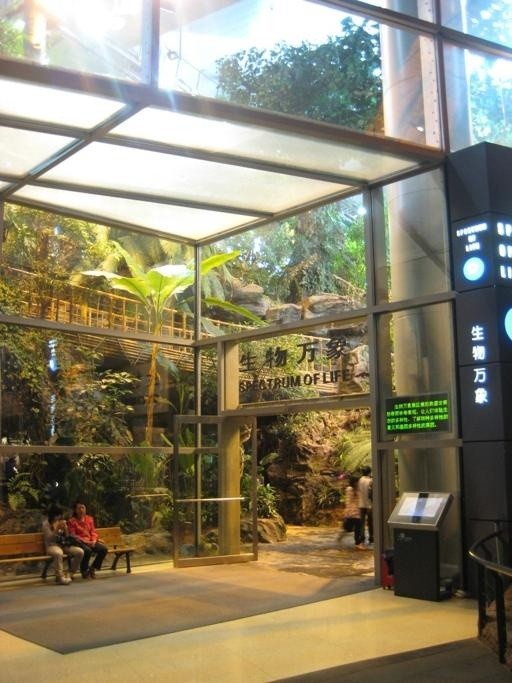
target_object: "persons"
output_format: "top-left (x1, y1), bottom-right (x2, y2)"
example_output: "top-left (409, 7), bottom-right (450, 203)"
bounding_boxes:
top-left (42, 505), bottom-right (86, 584)
top-left (356, 463), bottom-right (374, 551)
top-left (333, 475), bottom-right (367, 551)
top-left (69, 500), bottom-right (109, 581)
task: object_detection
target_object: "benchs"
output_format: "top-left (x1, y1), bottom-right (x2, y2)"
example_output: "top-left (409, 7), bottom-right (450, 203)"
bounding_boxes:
top-left (0, 526), bottom-right (136, 578)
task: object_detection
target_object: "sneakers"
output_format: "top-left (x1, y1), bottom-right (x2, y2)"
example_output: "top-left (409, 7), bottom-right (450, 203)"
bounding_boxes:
top-left (80, 569), bottom-right (90, 579)
top-left (336, 536), bottom-right (374, 550)
top-left (56, 575), bottom-right (73, 585)
top-left (66, 569), bottom-right (83, 581)
top-left (88, 566), bottom-right (98, 579)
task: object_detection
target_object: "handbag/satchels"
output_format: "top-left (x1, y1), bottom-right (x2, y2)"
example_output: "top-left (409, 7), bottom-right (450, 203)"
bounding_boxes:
top-left (55, 531), bottom-right (75, 547)
top-left (343, 516), bottom-right (362, 532)
